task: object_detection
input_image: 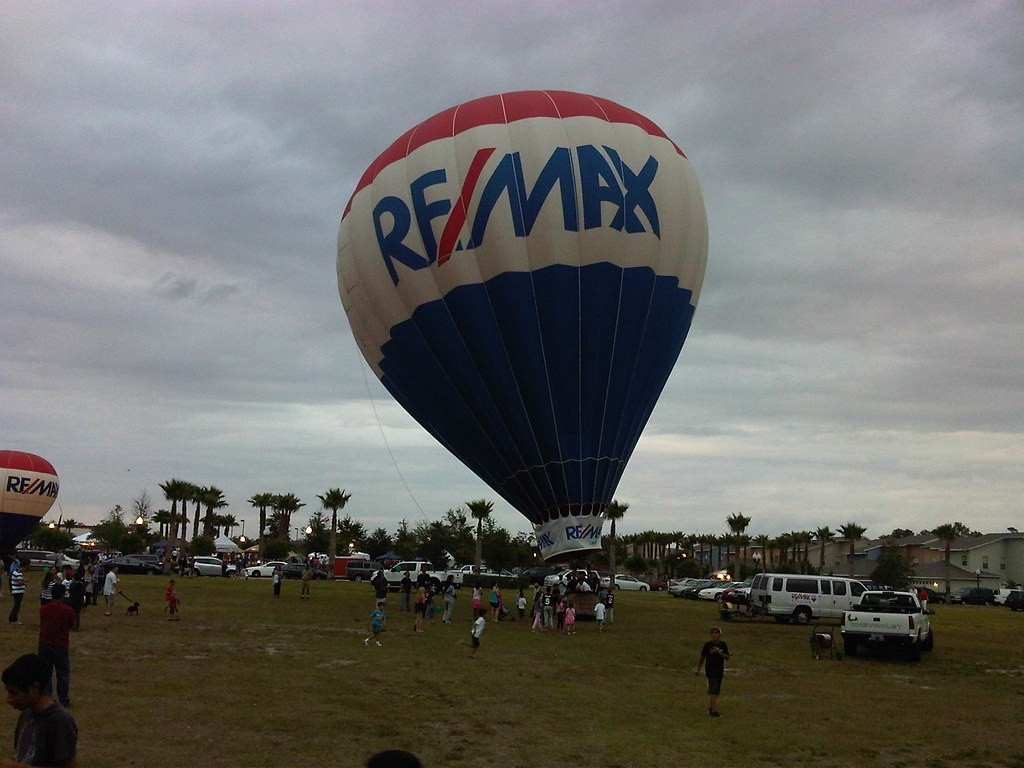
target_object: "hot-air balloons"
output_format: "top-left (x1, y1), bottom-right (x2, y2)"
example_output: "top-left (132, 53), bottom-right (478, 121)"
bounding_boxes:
top-left (0, 449), bottom-right (60, 572)
top-left (335, 89), bottom-right (710, 619)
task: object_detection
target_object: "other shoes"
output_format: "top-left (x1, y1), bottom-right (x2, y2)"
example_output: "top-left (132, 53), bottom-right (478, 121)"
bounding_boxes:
top-left (376, 642), bottom-right (382, 646)
top-left (364, 640), bottom-right (368, 645)
top-left (708, 708), bottom-right (721, 718)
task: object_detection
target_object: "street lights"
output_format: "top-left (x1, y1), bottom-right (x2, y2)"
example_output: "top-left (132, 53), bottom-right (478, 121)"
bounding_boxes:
top-left (753, 553), bottom-right (761, 571)
top-left (49, 523), bottom-right (55, 548)
top-left (241, 520), bottom-right (244, 550)
top-left (294, 528), bottom-right (298, 541)
top-left (976, 569), bottom-right (981, 588)
top-left (136, 516), bottom-right (143, 555)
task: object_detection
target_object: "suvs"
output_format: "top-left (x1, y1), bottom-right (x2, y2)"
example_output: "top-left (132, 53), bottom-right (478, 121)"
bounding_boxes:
top-left (345, 561), bottom-right (386, 581)
top-left (543, 568), bottom-right (600, 590)
top-left (184, 556), bottom-right (237, 578)
top-left (126, 555), bottom-right (161, 566)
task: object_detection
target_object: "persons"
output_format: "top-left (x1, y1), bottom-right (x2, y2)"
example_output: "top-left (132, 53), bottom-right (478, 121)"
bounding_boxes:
top-left (173, 549), bottom-right (194, 577)
top-left (0, 654), bottom-right (79, 768)
top-left (305, 566), bottom-right (312, 599)
top-left (388, 561), bottom-right (395, 569)
top-left (8, 553), bottom-right (20, 599)
top-left (222, 554), bottom-right (228, 577)
top-left (694, 626), bottom-right (730, 717)
top-left (38, 584), bottom-right (77, 707)
top-left (41, 549), bottom-right (119, 631)
top-left (910, 588), bottom-right (929, 604)
top-left (7, 561), bottom-right (30, 625)
top-left (364, 568), bottom-right (527, 657)
top-left (164, 579), bottom-right (180, 621)
top-left (291, 556), bottom-right (328, 566)
top-left (0, 559), bottom-right (4, 598)
top-left (272, 565), bottom-right (283, 599)
top-left (530, 561), bottom-right (614, 635)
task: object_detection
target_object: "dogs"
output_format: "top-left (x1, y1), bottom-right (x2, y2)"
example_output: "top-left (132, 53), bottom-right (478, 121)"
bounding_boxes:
top-left (125, 602), bottom-right (139, 616)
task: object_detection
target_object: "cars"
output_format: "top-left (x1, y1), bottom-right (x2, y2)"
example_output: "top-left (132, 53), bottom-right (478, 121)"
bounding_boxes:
top-left (62, 555), bottom-right (80, 571)
top-left (644, 579), bottom-right (667, 592)
top-left (101, 556), bottom-right (162, 576)
top-left (599, 573), bottom-right (650, 592)
top-left (279, 563), bottom-right (327, 581)
top-left (244, 561), bottom-right (287, 577)
top-left (668, 577), bottom-right (752, 603)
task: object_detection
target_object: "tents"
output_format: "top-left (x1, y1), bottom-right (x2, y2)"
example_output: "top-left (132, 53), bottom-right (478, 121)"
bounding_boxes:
top-left (213, 534), bottom-right (259, 552)
top-left (153, 540), bottom-right (168, 546)
top-left (376, 553), bottom-right (423, 560)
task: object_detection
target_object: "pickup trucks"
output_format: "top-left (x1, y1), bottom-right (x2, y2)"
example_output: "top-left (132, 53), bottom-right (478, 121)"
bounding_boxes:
top-left (838, 589), bottom-right (935, 659)
top-left (448, 564), bottom-right (502, 576)
top-left (517, 566), bottom-right (558, 586)
top-left (370, 561), bottom-right (464, 594)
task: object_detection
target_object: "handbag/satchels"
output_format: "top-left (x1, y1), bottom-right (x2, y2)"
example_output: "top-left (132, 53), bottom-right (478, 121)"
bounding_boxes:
top-left (489, 591), bottom-right (499, 603)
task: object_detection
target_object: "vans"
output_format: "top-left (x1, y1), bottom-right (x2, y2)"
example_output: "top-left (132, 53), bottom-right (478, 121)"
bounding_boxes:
top-left (18, 550), bottom-right (63, 570)
top-left (911, 586), bottom-right (1024, 612)
top-left (750, 573), bottom-right (869, 625)
